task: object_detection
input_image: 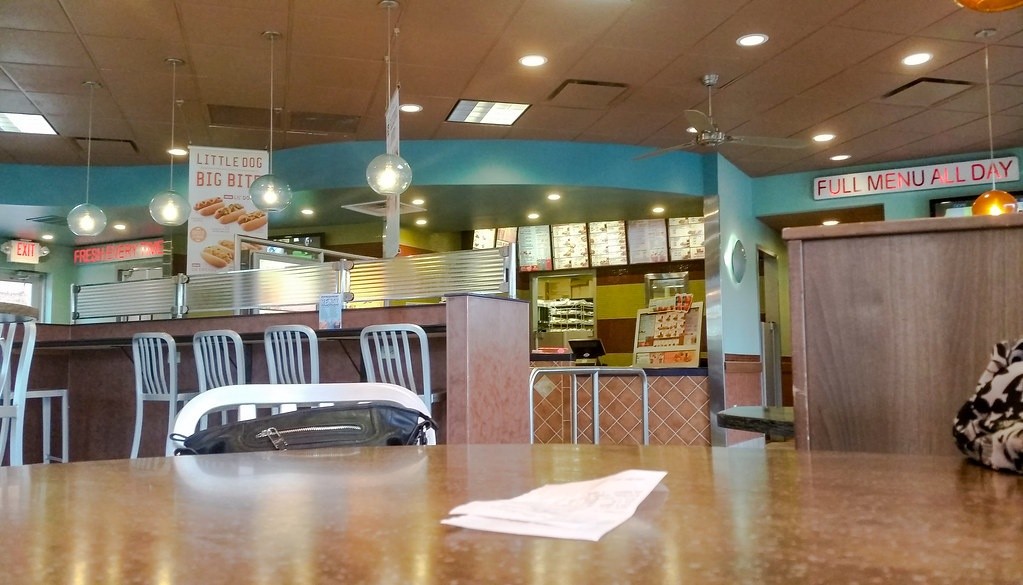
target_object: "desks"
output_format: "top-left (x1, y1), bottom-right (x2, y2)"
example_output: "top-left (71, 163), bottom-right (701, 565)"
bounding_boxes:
top-left (716, 405), bottom-right (795, 445)
top-left (0, 444), bottom-right (1023, 585)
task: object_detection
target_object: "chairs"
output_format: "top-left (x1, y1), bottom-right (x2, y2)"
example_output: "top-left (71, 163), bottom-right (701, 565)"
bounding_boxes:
top-left (130, 332), bottom-right (199, 459)
top-left (360, 323), bottom-right (445, 445)
top-left (193, 330), bottom-right (246, 431)
top-left (174, 382), bottom-right (436, 445)
top-left (0, 313), bottom-right (69, 466)
top-left (263, 325), bottom-right (319, 416)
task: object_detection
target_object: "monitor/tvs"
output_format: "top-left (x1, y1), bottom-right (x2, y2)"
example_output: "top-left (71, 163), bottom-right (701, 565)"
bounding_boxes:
top-left (266, 232), bottom-right (327, 263)
top-left (568, 337), bottom-right (606, 366)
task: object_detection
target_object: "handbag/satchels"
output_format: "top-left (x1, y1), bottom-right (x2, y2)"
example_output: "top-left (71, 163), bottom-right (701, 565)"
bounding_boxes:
top-left (170, 399), bottom-right (440, 455)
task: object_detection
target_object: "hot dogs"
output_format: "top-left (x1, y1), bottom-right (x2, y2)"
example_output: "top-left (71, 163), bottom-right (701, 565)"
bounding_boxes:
top-left (193, 196), bottom-right (268, 231)
top-left (201, 239), bottom-right (263, 267)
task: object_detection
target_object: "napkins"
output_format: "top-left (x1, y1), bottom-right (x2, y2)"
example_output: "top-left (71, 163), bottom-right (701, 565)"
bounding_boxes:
top-left (440, 469), bottom-right (669, 541)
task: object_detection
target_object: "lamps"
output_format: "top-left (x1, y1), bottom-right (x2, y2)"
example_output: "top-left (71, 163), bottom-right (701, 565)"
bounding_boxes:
top-left (67, 81), bottom-right (107, 237)
top-left (366, 0), bottom-right (413, 195)
top-left (249, 30), bottom-right (293, 212)
top-left (148, 58), bottom-right (192, 227)
top-left (971, 28), bottom-right (1018, 215)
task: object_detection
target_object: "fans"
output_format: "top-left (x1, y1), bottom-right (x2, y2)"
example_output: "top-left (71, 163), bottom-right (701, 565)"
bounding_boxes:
top-left (631, 73), bottom-right (808, 161)
